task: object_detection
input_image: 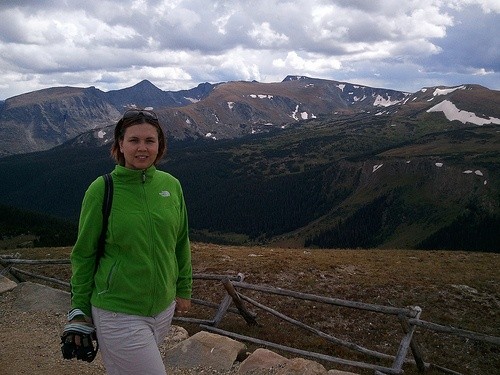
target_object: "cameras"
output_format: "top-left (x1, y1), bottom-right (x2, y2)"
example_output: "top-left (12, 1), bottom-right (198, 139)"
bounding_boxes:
top-left (61, 334), bottom-right (96, 362)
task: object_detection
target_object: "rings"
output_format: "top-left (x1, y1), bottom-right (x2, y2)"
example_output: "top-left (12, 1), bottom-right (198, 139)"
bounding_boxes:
top-left (184, 311), bottom-right (188, 313)
top-left (178, 310), bottom-right (182, 313)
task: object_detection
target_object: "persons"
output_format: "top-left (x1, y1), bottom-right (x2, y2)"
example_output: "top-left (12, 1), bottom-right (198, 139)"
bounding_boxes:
top-left (60, 108), bottom-right (193, 375)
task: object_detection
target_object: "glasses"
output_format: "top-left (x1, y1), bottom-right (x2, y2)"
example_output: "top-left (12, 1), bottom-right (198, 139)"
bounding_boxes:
top-left (122, 109), bottom-right (158, 120)
